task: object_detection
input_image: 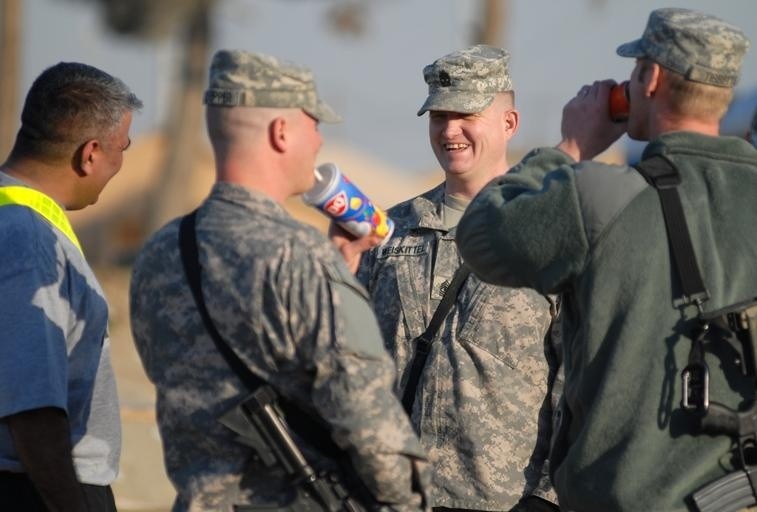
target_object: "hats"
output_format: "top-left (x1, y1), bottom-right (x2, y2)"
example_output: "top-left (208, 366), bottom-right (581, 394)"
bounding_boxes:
top-left (617, 8), bottom-right (750, 87)
top-left (417, 45), bottom-right (514, 116)
top-left (202, 48), bottom-right (343, 123)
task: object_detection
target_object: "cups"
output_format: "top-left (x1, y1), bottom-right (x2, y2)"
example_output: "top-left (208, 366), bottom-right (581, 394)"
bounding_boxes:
top-left (299, 162), bottom-right (395, 252)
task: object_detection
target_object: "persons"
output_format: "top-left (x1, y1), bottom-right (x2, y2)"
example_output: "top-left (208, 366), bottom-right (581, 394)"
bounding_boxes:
top-left (127, 45), bottom-right (436, 512)
top-left (355, 44), bottom-right (567, 509)
top-left (455, 10), bottom-right (757, 512)
top-left (0, 61), bottom-right (143, 512)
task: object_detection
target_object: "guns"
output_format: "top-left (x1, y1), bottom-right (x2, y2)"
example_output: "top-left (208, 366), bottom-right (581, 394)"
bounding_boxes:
top-left (677, 300), bottom-right (753, 512)
top-left (220, 386), bottom-right (368, 511)
